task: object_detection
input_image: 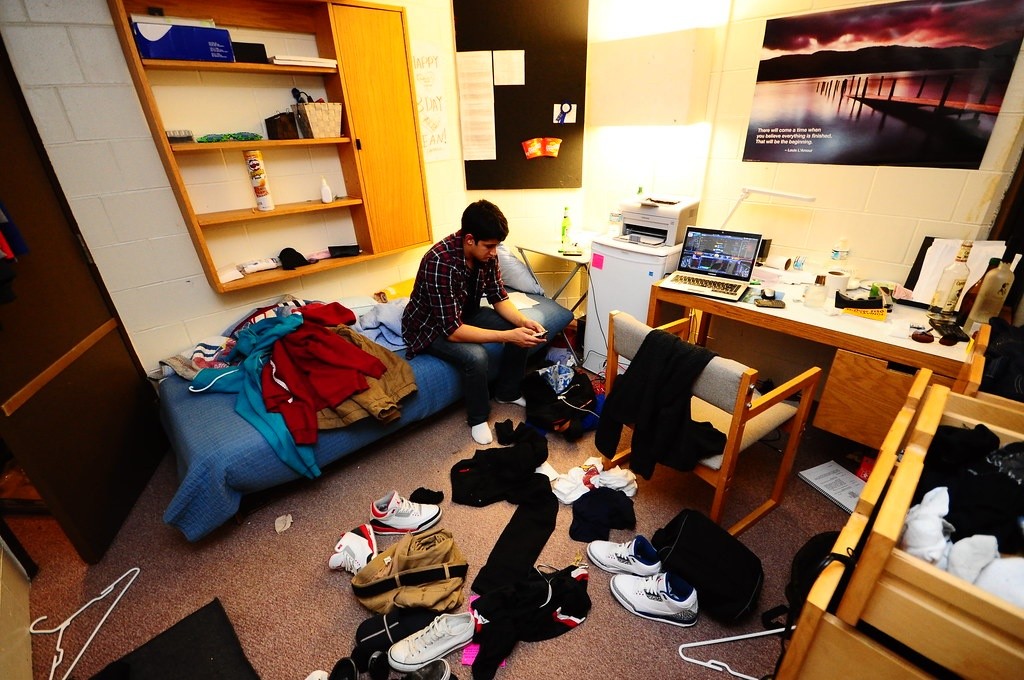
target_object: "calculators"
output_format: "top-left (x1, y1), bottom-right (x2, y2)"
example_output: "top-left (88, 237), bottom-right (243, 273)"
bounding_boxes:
top-left (930, 319), bottom-right (970, 342)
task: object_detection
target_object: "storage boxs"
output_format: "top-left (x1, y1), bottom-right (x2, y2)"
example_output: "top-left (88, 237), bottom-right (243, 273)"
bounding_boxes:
top-left (855, 454), bottom-right (876, 481)
top-left (132, 23), bottom-right (236, 62)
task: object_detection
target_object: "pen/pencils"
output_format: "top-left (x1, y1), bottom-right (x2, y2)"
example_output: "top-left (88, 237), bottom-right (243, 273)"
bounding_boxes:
top-left (749, 283), bottom-right (761, 285)
top-left (926, 329), bottom-right (933, 332)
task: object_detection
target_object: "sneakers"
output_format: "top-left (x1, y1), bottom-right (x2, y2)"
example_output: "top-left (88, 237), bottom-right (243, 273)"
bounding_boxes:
top-left (369, 491), bottom-right (443, 535)
top-left (610, 572), bottom-right (699, 627)
top-left (388, 613), bottom-right (475, 672)
top-left (328, 524), bottom-right (378, 576)
top-left (586, 534), bottom-right (663, 577)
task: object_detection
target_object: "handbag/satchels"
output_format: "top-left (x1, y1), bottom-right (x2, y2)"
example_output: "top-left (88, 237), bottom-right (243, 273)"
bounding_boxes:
top-left (525, 360), bottom-right (597, 441)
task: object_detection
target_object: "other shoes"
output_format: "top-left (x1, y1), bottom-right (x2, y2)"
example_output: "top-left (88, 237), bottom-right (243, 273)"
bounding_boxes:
top-left (404, 659), bottom-right (451, 680)
top-left (305, 670), bottom-right (328, 680)
top-left (328, 658), bottom-right (359, 680)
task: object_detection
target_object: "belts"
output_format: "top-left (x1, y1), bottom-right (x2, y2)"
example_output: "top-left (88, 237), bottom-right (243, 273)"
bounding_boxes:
top-left (352, 564), bottom-right (469, 598)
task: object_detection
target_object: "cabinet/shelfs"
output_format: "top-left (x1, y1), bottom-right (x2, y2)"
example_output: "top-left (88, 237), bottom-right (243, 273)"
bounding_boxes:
top-left (776, 367), bottom-right (1024, 680)
top-left (107, 0), bottom-right (433, 292)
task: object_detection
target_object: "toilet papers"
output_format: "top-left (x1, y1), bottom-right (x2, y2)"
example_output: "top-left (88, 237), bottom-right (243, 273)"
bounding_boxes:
top-left (824, 270), bottom-right (850, 298)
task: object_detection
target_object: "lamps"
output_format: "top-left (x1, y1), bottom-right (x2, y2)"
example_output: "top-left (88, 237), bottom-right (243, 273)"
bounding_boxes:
top-left (719, 188), bottom-right (816, 229)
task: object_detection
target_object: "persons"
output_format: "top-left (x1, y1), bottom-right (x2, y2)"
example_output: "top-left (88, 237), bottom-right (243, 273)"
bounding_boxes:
top-left (400, 201), bottom-right (546, 445)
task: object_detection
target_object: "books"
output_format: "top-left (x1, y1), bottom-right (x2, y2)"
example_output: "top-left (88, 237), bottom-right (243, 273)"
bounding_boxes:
top-left (799, 460), bottom-right (866, 514)
top-left (268, 55), bottom-right (338, 68)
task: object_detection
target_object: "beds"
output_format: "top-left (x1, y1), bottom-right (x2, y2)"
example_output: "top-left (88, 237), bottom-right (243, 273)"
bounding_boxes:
top-left (159, 281), bottom-right (575, 541)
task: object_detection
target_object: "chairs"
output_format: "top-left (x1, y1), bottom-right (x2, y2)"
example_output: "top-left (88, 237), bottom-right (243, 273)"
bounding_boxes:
top-left (601, 311), bottom-right (822, 537)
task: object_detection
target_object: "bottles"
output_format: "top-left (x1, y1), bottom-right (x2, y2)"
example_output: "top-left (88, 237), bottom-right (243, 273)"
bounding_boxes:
top-left (243, 150), bottom-right (275, 211)
top-left (804, 275), bottom-right (829, 309)
top-left (926, 241), bottom-right (971, 320)
top-left (320, 177), bottom-right (332, 204)
top-left (963, 247), bottom-right (1016, 334)
top-left (956, 257), bottom-right (999, 325)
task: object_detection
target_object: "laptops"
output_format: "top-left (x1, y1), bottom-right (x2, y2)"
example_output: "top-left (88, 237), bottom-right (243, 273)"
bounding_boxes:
top-left (659, 226), bottom-right (763, 301)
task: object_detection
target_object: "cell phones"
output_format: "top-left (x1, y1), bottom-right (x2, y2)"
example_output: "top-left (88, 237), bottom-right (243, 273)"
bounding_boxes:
top-left (754, 299), bottom-right (786, 308)
top-left (532, 331), bottom-right (549, 339)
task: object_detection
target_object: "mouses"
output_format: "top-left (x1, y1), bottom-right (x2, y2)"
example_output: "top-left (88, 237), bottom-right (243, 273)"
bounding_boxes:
top-left (761, 289), bottom-right (776, 300)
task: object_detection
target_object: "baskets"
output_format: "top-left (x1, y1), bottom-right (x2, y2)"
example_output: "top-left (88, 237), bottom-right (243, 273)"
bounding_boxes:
top-left (290, 102), bottom-right (342, 139)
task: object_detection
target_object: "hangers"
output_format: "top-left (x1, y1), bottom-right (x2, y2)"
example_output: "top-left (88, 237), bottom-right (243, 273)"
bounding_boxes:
top-left (678, 626), bottom-right (797, 680)
top-left (29, 566), bottom-right (140, 680)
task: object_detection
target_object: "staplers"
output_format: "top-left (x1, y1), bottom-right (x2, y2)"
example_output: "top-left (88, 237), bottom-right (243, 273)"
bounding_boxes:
top-left (869, 282), bottom-right (894, 312)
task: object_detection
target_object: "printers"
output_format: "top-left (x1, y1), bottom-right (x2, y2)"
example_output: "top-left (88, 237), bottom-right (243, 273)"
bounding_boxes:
top-left (609, 197), bottom-right (700, 248)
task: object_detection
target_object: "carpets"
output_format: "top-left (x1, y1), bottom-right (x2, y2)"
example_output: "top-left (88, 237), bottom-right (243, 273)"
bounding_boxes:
top-left (89, 599), bottom-right (261, 680)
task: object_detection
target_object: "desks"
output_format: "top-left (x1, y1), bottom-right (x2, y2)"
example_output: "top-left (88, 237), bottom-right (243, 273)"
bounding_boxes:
top-left (647, 268), bottom-right (992, 450)
top-left (514, 242), bottom-right (590, 367)
